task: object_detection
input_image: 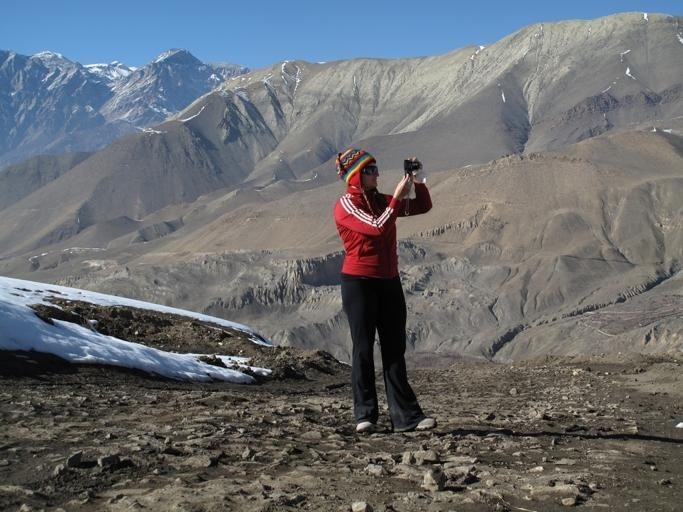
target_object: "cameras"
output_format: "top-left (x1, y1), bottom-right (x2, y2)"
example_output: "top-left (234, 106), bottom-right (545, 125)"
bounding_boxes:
top-left (404, 159), bottom-right (420, 171)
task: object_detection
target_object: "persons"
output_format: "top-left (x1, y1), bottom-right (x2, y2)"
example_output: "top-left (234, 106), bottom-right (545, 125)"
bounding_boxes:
top-left (334, 146), bottom-right (438, 432)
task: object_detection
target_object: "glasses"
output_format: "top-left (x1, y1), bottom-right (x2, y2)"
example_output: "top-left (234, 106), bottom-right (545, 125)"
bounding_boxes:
top-left (361, 165), bottom-right (380, 176)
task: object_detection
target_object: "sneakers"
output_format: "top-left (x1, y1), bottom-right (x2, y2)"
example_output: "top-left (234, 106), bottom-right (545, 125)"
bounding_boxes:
top-left (416, 418), bottom-right (437, 430)
top-left (356, 421), bottom-right (374, 432)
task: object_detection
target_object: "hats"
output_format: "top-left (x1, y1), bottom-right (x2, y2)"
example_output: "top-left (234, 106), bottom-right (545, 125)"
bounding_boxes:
top-left (335, 148), bottom-right (377, 188)
